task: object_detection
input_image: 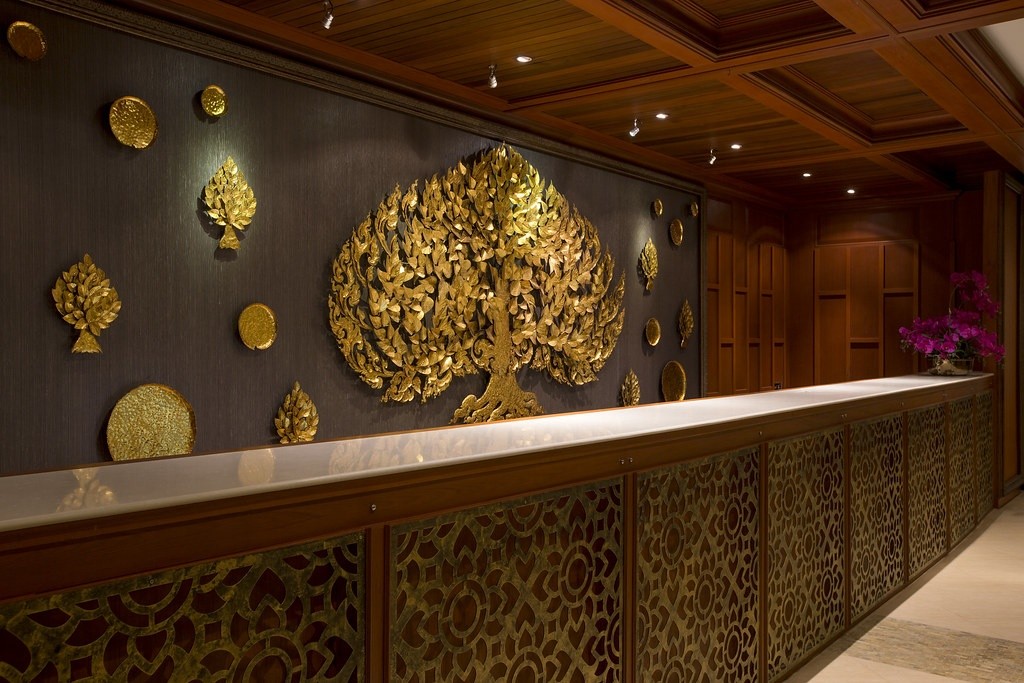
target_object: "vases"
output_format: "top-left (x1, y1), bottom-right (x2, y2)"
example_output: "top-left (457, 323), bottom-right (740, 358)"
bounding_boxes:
top-left (929, 359), bottom-right (971, 375)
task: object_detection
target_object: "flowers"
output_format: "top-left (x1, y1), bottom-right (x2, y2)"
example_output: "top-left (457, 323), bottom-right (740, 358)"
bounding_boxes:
top-left (900, 272), bottom-right (1006, 364)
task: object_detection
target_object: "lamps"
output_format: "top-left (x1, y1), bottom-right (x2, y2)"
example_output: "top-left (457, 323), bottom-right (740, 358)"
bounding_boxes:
top-left (488, 64), bottom-right (498, 88)
top-left (629, 118), bottom-right (639, 137)
top-left (320, 0), bottom-right (334, 30)
top-left (708, 148), bottom-right (717, 164)
